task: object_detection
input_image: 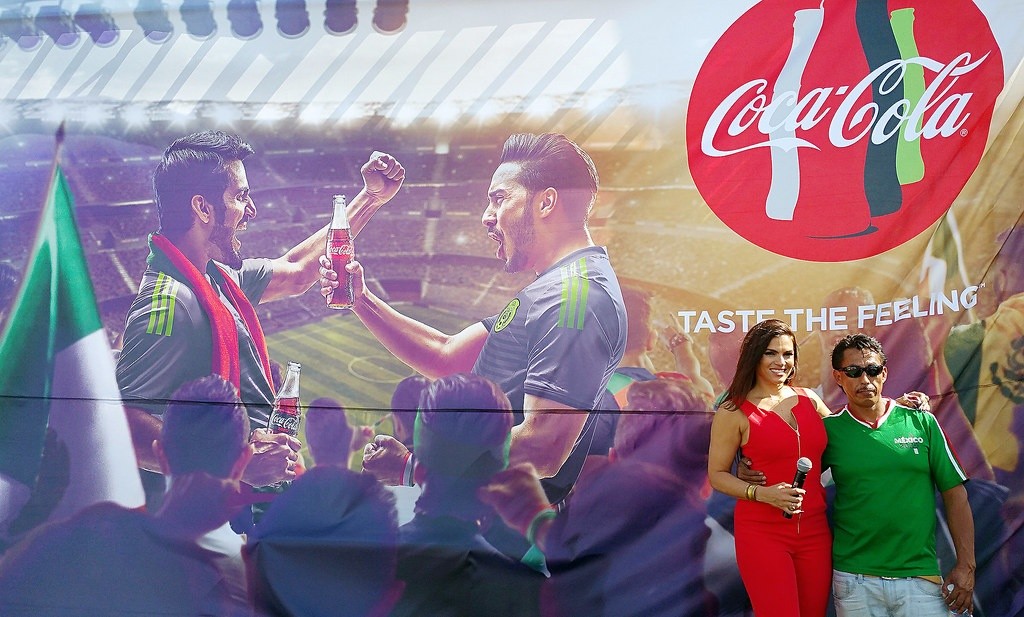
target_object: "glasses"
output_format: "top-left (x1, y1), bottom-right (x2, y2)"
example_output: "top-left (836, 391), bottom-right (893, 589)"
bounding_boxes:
top-left (839, 364), bottom-right (885, 377)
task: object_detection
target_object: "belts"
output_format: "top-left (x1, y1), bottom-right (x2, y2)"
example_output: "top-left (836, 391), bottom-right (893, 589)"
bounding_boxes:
top-left (863, 574), bottom-right (942, 584)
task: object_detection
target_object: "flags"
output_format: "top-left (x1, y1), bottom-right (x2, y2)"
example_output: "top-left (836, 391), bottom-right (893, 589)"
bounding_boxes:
top-left (0, 119), bottom-right (147, 541)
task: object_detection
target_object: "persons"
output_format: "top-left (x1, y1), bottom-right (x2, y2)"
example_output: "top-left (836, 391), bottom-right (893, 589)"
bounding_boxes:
top-left (0, 132), bottom-right (1024, 617)
top-left (737, 331), bottom-right (976, 617)
top-left (705, 321), bottom-right (931, 617)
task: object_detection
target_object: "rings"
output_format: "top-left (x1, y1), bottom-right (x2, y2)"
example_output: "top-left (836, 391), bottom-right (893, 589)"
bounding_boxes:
top-left (791, 502), bottom-right (797, 511)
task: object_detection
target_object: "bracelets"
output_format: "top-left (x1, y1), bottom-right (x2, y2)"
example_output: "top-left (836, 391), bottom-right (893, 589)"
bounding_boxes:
top-left (525, 505), bottom-right (556, 551)
top-left (401, 451), bottom-right (416, 489)
top-left (745, 483), bottom-right (759, 502)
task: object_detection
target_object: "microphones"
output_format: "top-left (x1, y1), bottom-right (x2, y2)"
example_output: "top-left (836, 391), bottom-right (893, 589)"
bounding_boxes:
top-left (784, 457), bottom-right (813, 519)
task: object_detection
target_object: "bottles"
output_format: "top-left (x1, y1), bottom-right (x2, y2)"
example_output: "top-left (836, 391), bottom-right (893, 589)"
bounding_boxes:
top-left (266, 360), bottom-right (303, 487)
top-left (325, 194), bottom-right (355, 309)
top-left (947, 583), bottom-right (973, 617)
